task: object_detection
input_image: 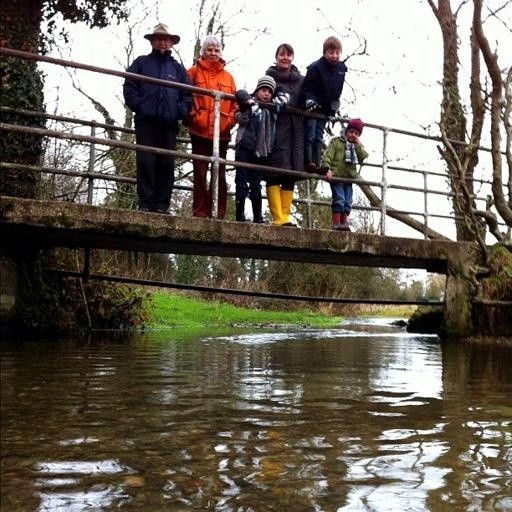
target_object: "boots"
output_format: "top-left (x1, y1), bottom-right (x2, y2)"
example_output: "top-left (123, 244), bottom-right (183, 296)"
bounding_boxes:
top-left (305, 142), bottom-right (329, 175)
top-left (332, 212), bottom-right (350, 231)
top-left (235, 192), bottom-right (266, 223)
top-left (266, 185), bottom-right (297, 227)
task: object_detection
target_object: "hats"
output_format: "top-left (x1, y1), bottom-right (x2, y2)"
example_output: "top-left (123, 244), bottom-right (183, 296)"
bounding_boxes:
top-left (255, 76), bottom-right (277, 93)
top-left (347, 118), bottom-right (364, 133)
top-left (144, 23), bottom-right (181, 44)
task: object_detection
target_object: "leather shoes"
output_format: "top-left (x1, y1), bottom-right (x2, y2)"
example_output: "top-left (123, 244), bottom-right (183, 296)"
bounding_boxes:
top-left (139, 207), bottom-right (170, 214)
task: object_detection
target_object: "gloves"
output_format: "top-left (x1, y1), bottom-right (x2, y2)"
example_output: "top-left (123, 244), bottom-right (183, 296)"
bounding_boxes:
top-left (306, 99), bottom-right (322, 113)
top-left (251, 102), bottom-right (262, 122)
top-left (272, 95), bottom-right (288, 109)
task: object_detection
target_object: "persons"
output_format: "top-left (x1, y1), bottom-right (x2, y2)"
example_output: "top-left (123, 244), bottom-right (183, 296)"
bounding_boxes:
top-left (124, 23), bottom-right (194, 213)
top-left (320, 118), bottom-right (368, 229)
top-left (237, 75), bottom-right (292, 225)
top-left (265, 44), bottom-right (305, 227)
top-left (186, 35), bottom-right (237, 221)
top-left (301, 36), bottom-right (348, 179)
